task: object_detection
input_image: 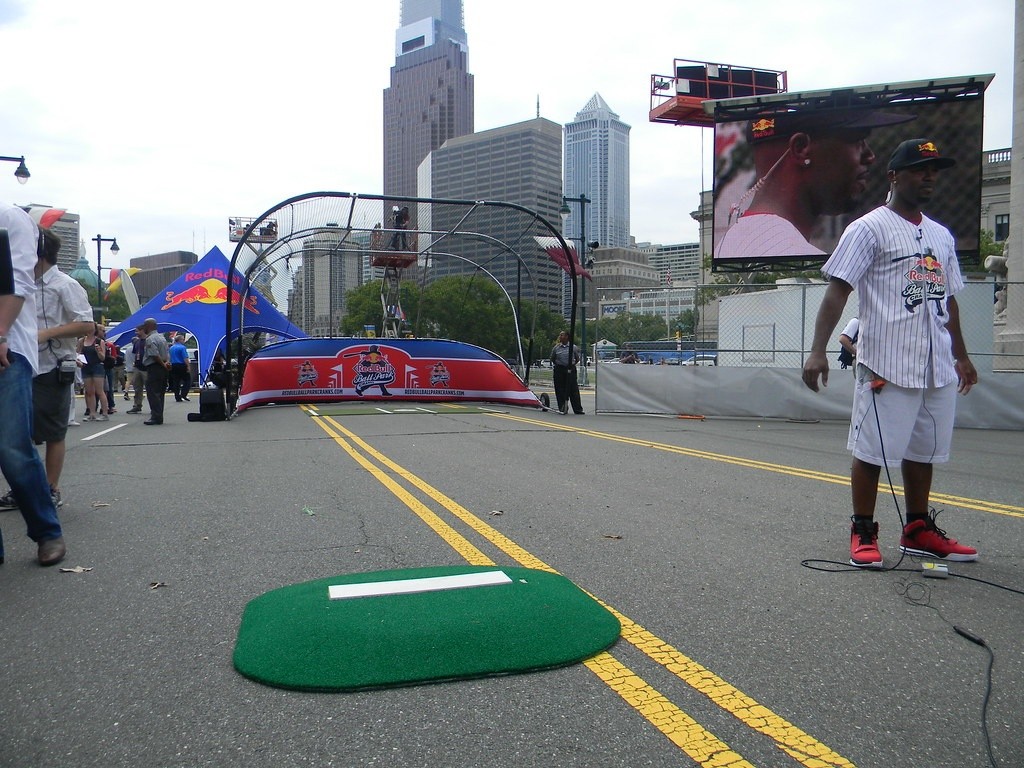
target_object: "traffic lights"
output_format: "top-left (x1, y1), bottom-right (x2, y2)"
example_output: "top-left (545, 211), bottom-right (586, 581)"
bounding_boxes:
top-left (676, 331), bottom-right (680, 339)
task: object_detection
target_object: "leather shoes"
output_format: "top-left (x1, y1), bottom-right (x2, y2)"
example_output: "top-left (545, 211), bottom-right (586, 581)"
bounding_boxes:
top-left (37, 534), bottom-right (66, 565)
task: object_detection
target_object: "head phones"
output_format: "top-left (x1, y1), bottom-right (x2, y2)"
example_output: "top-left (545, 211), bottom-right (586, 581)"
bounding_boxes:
top-left (38, 228), bottom-right (47, 258)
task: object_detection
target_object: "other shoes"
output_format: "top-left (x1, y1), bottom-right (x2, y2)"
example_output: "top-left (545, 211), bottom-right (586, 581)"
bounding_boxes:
top-left (175, 398), bottom-right (183, 401)
top-left (68, 420), bottom-right (80, 426)
top-left (144, 419), bottom-right (163, 425)
top-left (181, 395), bottom-right (190, 401)
top-left (0, 489), bottom-right (19, 511)
top-left (576, 411), bottom-right (585, 414)
top-left (48, 483), bottom-right (63, 509)
top-left (124, 393), bottom-right (130, 400)
top-left (83, 406), bottom-right (117, 421)
top-left (127, 407), bottom-right (142, 414)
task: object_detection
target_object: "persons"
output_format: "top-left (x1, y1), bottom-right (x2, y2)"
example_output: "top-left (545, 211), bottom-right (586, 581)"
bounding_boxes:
top-left (0, 204), bottom-right (66, 565)
top-left (841, 313), bottom-right (865, 380)
top-left (394, 207), bottom-right (409, 250)
top-left (551, 330), bottom-right (587, 416)
top-left (1, 223), bottom-right (97, 508)
top-left (214, 347), bottom-right (226, 363)
top-left (713, 116), bottom-right (875, 258)
top-left (169, 335), bottom-right (193, 402)
top-left (267, 222), bottom-right (277, 235)
top-left (801, 140), bottom-right (980, 568)
top-left (620, 345), bottom-right (641, 364)
top-left (77, 319), bottom-right (169, 425)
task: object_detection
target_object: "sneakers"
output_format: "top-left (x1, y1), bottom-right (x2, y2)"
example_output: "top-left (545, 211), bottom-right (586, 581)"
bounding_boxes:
top-left (901, 506), bottom-right (978, 561)
top-left (850, 514), bottom-right (884, 568)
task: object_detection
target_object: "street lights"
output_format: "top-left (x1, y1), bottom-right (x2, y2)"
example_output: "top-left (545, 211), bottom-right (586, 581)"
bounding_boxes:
top-left (557, 192), bottom-right (590, 387)
top-left (90, 233), bottom-right (120, 327)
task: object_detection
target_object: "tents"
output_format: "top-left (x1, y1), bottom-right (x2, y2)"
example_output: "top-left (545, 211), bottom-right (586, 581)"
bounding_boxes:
top-left (103, 245), bottom-right (309, 389)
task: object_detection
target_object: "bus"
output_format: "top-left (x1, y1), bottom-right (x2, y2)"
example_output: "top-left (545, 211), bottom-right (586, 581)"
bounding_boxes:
top-left (618, 339), bottom-right (718, 364)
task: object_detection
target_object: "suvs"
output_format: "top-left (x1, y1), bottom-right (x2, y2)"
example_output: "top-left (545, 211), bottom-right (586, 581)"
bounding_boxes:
top-left (681, 354), bottom-right (717, 366)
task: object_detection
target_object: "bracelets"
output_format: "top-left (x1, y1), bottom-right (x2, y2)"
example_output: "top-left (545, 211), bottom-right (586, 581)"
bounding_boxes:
top-left (0, 336), bottom-right (8, 343)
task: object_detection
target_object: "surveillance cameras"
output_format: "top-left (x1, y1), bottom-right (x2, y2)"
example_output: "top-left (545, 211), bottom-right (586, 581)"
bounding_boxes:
top-left (587, 240), bottom-right (599, 248)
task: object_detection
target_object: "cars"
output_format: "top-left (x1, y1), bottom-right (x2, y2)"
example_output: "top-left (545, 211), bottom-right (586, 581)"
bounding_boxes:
top-left (185, 348), bottom-right (199, 384)
top-left (654, 357), bottom-right (678, 365)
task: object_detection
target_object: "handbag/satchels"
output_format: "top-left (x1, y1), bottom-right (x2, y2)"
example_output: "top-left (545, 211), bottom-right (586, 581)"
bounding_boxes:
top-left (837, 317), bottom-right (861, 369)
top-left (58, 360), bottom-right (76, 383)
top-left (104, 350), bottom-right (114, 370)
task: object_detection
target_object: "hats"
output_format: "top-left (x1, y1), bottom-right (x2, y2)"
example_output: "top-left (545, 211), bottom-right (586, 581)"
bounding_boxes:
top-left (746, 103), bottom-right (918, 142)
top-left (888, 138), bottom-right (955, 169)
